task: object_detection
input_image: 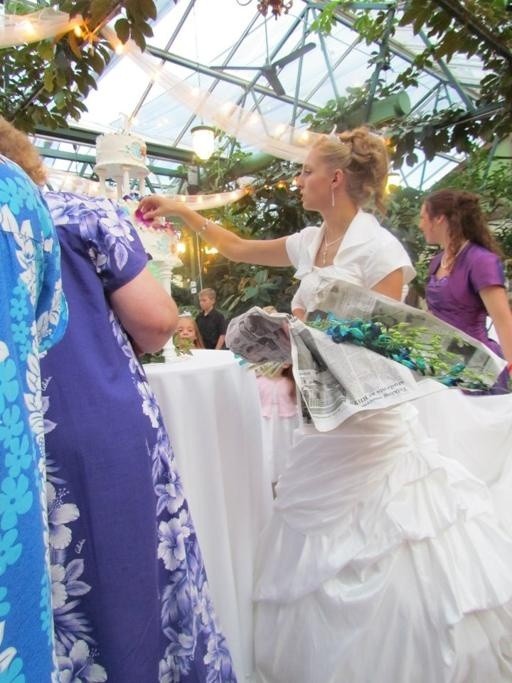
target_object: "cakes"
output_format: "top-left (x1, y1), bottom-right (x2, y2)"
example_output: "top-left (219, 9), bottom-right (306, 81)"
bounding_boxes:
top-left (95, 132), bottom-right (148, 164)
top-left (119, 198), bottom-right (177, 256)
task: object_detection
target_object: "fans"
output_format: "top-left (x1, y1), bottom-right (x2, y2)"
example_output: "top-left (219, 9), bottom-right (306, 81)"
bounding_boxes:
top-left (208, 1), bottom-right (318, 98)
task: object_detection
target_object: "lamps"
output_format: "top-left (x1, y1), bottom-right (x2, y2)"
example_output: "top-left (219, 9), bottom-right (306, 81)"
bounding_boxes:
top-left (187, 1), bottom-right (218, 162)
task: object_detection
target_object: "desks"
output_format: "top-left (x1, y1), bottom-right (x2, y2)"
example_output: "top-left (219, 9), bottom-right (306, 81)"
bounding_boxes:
top-left (136, 348), bottom-right (273, 683)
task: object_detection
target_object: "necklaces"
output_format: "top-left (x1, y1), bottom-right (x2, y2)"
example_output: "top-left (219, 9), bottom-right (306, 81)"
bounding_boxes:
top-left (322, 233), bottom-right (342, 264)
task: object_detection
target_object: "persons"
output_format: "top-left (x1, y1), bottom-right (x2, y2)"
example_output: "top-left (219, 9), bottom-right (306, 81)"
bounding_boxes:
top-left (416, 188), bottom-right (512, 396)
top-left (0, 114), bottom-right (236, 682)
top-left (0, 152), bottom-right (71, 682)
top-left (136, 126), bottom-right (512, 683)
top-left (172, 287), bottom-right (298, 494)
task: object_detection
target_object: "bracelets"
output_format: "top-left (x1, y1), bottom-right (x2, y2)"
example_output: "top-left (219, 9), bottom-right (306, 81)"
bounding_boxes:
top-left (196, 217), bottom-right (209, 238)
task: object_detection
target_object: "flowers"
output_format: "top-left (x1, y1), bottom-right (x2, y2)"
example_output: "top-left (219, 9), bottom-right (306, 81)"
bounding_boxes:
top-left (235, 310), bottom-right (500, 393)
